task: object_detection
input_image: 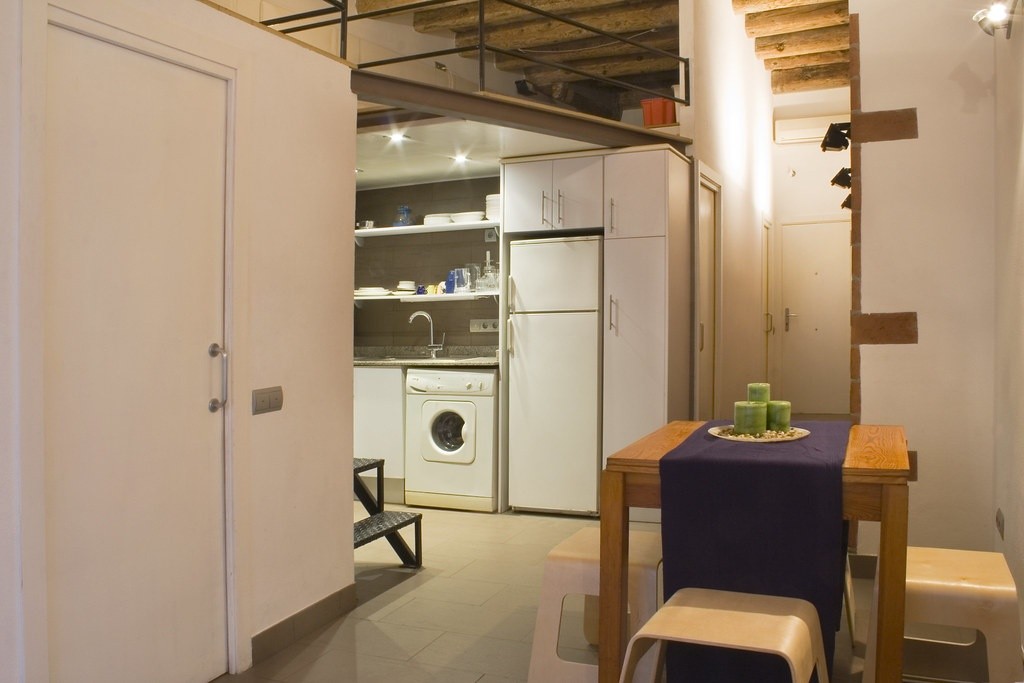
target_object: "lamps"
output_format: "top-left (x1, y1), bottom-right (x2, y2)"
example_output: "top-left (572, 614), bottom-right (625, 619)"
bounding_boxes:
top-left (973, 0), bottom-right (1018, 39)
top-left (821, 122), bottom-right (852, 209)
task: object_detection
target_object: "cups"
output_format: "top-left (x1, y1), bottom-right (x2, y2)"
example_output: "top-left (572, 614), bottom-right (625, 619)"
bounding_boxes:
top-left (748, 383), bottom-right (770, 400)
top-left (767, 400), bottom-right (791, 433)
top-left (733, 402), bottom-right (767, 434)
top-left (453, 263), bottom-right (499, 293)
top-left (397, 281), bottom-right (415, 290)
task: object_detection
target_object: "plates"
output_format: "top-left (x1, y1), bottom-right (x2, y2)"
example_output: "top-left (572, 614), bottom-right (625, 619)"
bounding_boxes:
top-left (391, 292), bottom-right (416, 296)
top-left (708, 424), bottom-right (809, 442)
top-left (354, 287), bottom-right (392, 295)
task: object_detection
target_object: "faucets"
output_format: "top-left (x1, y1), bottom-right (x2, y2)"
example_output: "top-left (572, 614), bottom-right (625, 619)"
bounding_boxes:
top-left (408, 310), bottom-right (447, 359)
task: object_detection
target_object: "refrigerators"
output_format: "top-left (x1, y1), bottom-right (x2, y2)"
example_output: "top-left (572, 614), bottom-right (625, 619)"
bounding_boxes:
top-left (502, 234), bottom-right (604, 518)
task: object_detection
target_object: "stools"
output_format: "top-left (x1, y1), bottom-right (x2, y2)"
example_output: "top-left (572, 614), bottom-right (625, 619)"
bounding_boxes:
top-left (618, 586), bottom-right (831, 682)
top-left (862, 545), bottom-right (1023, 683)
top-left (529, 527), bottom-right (662, 683)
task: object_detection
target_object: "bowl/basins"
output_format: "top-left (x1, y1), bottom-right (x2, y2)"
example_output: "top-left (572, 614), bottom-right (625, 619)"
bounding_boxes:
top-left (358, 221), bottom-right (378, 228)
top-left (423, 194), bottom-right (500, 226)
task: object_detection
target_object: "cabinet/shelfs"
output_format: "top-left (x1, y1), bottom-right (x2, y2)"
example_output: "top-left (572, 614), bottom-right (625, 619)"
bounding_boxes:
top-left (353, 163), bottom-right (502, 302)
top-left (500, 144), bottom-right (691, 522)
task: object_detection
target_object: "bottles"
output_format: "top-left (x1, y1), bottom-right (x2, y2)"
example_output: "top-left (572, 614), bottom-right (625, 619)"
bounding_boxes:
top-left (392, 202), bottom-right (415, 226)
top-left (417, 270), bottom-right (453, 295)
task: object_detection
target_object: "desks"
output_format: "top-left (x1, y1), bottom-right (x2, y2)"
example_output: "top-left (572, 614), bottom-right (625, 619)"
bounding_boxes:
top-left (598, 414), bottom-right (910, 683)
